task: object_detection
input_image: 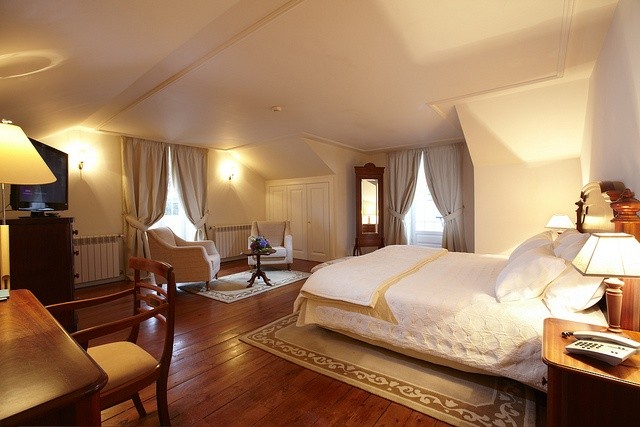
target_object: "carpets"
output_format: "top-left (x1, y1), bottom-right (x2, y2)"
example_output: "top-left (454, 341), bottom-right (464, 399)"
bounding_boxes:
top-left (177, 267), bottom-right (310, 304)
top-left (239, 311), bottom-right (538, 426)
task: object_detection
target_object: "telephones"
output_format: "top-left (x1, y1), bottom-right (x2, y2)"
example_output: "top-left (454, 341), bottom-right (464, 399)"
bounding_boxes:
top-left (562, 331), bottom-right (640, 366)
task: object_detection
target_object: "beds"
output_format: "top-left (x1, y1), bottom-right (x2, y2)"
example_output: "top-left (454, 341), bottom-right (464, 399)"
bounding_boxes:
top-left (292, 180), bottom-right (640, 394)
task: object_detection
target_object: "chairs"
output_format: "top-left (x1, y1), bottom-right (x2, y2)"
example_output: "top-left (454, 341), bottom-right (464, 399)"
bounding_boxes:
top-left (46, 257), bottom-right (175, 427)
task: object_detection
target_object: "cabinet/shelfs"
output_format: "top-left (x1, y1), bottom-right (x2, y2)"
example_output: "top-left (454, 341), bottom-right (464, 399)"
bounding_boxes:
top-left (265, 174), bottom-right (335, 263)
top-left (1, 217), bottom-right (78, 333)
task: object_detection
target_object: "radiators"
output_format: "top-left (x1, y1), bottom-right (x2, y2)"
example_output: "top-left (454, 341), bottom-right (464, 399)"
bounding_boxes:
top-left (213, 224), bottom-right (252, 260)
top-left (73, 233), bottom-right (124, 286)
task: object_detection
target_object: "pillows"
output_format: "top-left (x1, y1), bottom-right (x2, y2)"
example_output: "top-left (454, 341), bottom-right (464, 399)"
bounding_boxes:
top-left (506, 230), bottom-right (556, 261)
top-left (546, 227), bottom-right (607, 314)
top-left (494, 244), bottom-right (567, 303)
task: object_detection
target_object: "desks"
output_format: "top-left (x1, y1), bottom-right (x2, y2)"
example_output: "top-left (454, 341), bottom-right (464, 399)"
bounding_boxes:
top-left (542, 317), bottom-right (640, 427)
top-left (0, 286), bottom-right (109, 427)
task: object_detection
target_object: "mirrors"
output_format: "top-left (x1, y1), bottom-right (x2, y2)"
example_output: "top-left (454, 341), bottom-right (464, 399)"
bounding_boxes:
top-left (354, 163), bottom-right (386, 256)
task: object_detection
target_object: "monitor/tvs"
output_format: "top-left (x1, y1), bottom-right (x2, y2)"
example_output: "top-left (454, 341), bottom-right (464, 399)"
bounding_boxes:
top-left (10, 136), bottom-right (69, 218)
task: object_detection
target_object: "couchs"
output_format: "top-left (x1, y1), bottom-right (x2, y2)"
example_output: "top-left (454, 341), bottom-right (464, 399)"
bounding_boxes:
top-left (146, 226), bottom-right (221, 297)
top-left (247, 219), bottom-right (294, 271)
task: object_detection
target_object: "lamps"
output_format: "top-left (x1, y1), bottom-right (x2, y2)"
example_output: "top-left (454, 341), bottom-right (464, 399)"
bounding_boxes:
top-left (0, 115), bottom-right (58, 302)
top-left (545, 213), bottom-right (574, 236)
top-left (571, 230), bottom-right (639, 334)
